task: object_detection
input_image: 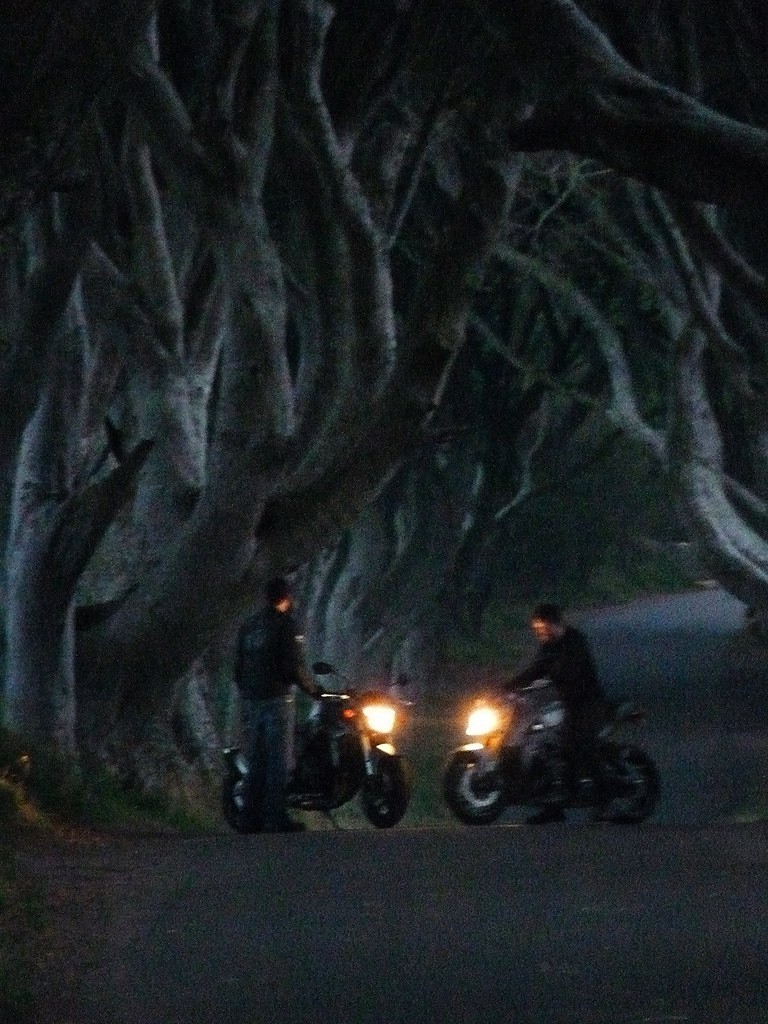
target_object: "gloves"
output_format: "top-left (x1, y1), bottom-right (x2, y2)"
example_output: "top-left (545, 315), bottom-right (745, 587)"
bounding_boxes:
top-left (314, 684), bottom-right (325, 701)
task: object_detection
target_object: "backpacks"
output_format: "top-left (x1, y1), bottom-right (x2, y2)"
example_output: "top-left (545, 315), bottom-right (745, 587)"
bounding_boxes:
top-left (239, 616), bottom-right (283, 695)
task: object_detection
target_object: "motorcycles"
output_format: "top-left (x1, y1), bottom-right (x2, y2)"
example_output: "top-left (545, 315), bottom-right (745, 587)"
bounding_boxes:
top-left (442, 682), bottom-right (663, 828)
top-left (218, 662), bottom-right (419, 831)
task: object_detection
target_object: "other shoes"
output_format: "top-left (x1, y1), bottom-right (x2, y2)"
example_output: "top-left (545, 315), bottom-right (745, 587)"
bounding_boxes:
top-left (527, 806), bottom-right (563, 824)
top-left (264, 810), bottom-right (307, 834)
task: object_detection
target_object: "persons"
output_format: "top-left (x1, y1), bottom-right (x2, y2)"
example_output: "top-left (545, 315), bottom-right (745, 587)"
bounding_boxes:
top-left (503, 605), bottom-right (607, 823)
top-left (234, 580), bottom-right (326, 827)
